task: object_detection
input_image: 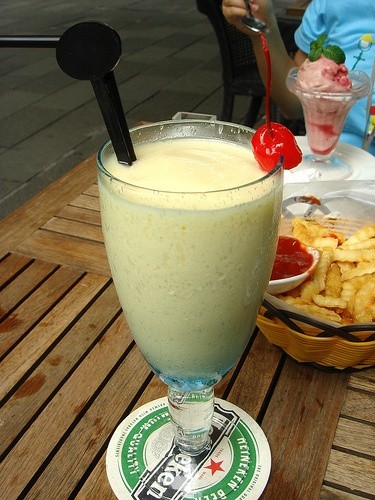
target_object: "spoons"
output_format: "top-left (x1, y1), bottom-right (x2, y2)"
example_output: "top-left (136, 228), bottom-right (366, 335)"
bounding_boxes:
top-left (240, 0), bottom-right (268, 33)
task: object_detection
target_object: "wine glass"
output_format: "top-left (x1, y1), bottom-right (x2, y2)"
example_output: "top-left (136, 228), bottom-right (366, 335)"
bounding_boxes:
top-left (95, 119), bottom-right (284, 500)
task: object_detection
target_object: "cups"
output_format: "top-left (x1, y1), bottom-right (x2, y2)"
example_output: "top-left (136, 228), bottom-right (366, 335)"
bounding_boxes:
top-left (285, 68), bottom-right (372, 181)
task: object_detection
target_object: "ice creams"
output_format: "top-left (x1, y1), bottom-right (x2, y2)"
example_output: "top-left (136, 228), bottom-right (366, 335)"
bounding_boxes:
top-left (296, 53), bottom-right (353, 156)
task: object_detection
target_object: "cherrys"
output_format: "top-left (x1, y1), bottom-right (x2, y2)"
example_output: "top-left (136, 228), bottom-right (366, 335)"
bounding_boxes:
top-left (250, 32), bottom-right (302, 172)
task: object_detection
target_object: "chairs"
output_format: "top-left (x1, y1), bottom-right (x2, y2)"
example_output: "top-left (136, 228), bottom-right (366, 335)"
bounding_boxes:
top-left (196, 0), bottom-right (301, 128)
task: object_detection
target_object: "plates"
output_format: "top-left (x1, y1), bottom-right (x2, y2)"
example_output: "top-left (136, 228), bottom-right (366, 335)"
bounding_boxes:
top-left (265, 247), bottom-right (319, 294)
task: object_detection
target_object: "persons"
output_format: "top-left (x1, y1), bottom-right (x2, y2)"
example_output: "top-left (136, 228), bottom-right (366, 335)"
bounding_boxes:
top-left (221, 0), bottom-right (375, 157)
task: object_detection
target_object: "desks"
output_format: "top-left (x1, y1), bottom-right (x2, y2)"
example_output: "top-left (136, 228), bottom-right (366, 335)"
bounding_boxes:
top-left (0, 120), bottom-right (375, 500)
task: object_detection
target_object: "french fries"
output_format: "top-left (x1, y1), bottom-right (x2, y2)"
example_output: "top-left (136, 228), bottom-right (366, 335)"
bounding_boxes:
top-left (259, 215), bottom-right (375, 329)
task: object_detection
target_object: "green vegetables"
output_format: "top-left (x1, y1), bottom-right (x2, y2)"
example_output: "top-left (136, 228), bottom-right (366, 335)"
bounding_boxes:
top-left (308, 32), bottom-right (346, 64)
